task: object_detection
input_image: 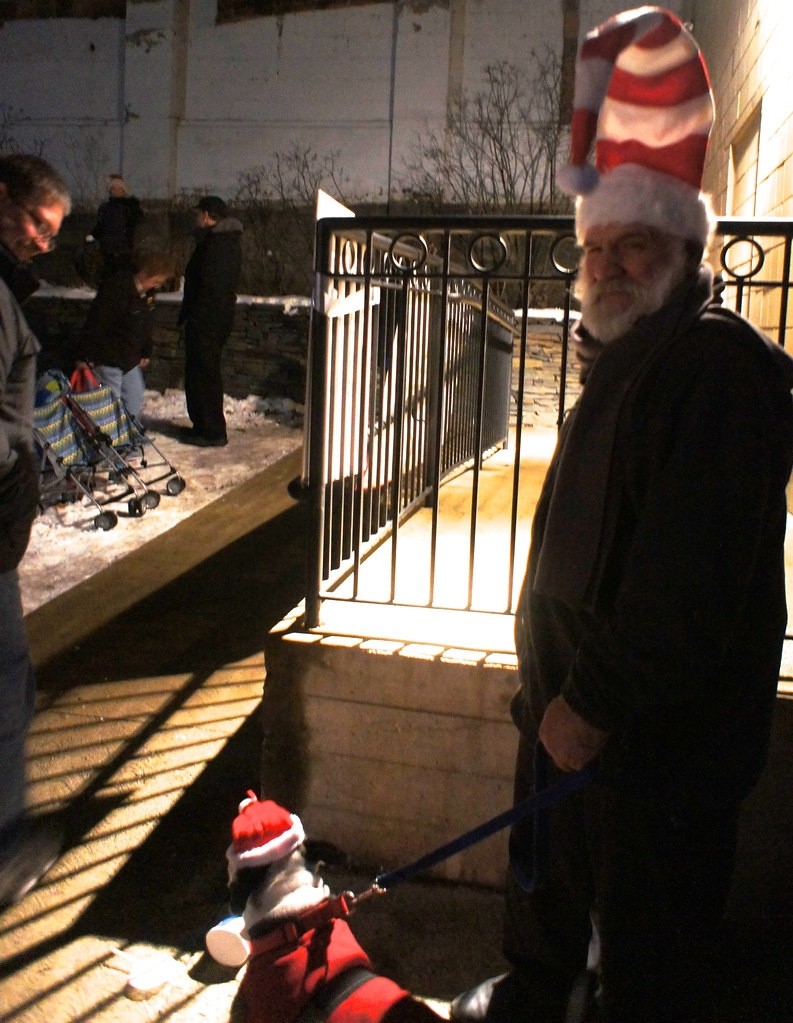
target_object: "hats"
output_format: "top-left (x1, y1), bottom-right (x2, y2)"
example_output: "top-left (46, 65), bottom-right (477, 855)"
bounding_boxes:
top-left (555, 5), bottom-right (715, 244)
top-left (105, 174), bottom-right (128, 194)
top-left (224, 795), bottom-right (306, 885)
top-left (193, 195), bottom-right (226, 219)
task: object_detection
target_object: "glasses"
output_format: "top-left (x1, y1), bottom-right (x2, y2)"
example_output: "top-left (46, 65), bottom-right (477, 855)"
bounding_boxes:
top-left (11, 194), bottom-right (58, 252)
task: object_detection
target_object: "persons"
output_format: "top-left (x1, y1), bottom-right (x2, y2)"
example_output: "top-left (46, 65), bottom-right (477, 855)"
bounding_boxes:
top-left (79, 250), bottom-right (174, 441)
top-left (96, 175), bottom-right (144, 265)
top-left (0, 152), bottom-right (73, 895)
top-left (507, 169), bottom-right (792, 1023)
top-left (176, 196), bottom-right (242, 449)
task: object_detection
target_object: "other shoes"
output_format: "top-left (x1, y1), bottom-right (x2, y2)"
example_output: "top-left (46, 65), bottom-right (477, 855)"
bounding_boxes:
top-left (181, 426), bottom-right (227, 447)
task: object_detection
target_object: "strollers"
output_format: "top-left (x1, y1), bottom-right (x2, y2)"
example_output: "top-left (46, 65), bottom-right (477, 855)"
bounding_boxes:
top-left (29, 375), bottom-right (146, 531)
top-left (59, 360), bottom-right (186, 510)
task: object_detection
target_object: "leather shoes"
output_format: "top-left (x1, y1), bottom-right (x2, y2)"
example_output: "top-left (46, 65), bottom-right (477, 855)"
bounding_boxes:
top-left (450, 967), bottom-right (552, 1023)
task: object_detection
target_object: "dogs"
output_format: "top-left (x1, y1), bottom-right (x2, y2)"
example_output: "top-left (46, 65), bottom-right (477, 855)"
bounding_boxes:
top-left (228, 791), bottom-right (446, 1023)
